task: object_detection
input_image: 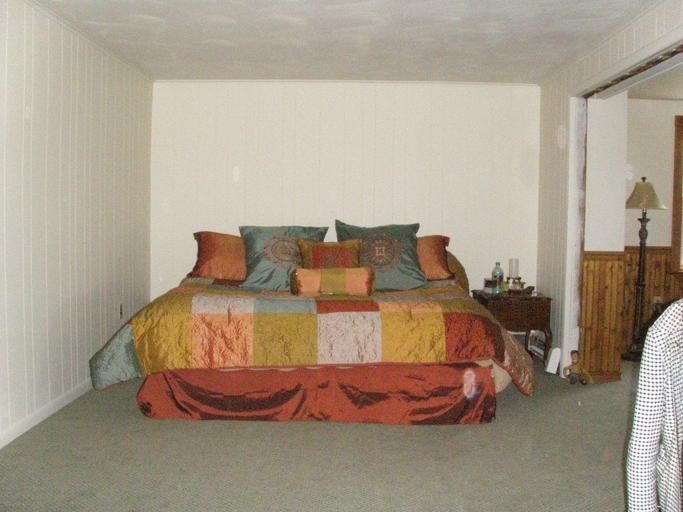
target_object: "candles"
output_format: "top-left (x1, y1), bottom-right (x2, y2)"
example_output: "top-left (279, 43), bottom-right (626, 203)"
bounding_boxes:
top-left (508, 257), bottom-right (518, 278)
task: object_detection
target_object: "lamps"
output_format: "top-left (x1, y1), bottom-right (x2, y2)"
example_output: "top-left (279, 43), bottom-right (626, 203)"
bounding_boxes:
top-left (620, 176), bottom-right (669, 363)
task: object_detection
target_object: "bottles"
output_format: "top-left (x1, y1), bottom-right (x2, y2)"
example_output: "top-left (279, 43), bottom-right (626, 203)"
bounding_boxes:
top-left (492, 262), bottom-right (503, 289)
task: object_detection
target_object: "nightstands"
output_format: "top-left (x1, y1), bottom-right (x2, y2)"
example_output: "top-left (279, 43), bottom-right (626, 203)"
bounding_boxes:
top-left (469, 289), bottom-right (553, 365)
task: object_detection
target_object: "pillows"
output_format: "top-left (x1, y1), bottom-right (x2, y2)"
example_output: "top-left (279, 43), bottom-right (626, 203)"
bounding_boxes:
top-left (293, 237), bottom-right (362, 268)
top-left (185, 231), bottom-right (247, 282)
top-left (288, 267), bottom-right (374, 297)
top-left (415, 235), bottom-right (455, 282)
top-left (334, 219), bottom-right (427, 292)
top-left (235, 225), bottom-right (329, 292)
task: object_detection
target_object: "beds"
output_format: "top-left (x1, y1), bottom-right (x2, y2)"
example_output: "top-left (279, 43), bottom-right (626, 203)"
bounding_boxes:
top-left (86, 249), bottom-right (534, 427)
